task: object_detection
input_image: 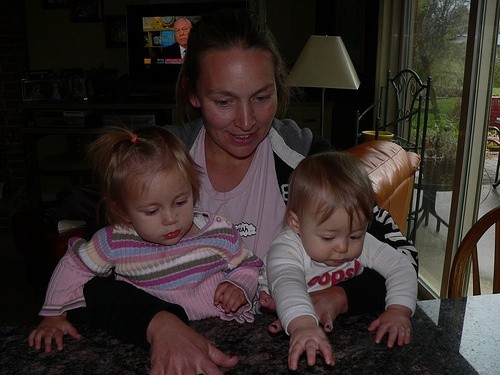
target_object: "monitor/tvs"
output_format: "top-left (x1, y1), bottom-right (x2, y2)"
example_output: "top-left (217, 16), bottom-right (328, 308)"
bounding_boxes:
top-left (126, 2), bottom-right (215, 80)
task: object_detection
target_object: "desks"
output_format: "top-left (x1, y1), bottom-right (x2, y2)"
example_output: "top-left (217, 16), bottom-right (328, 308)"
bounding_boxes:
top-left (0, 293), bottom-right (500, 375)
top-left (412, 174), bottom-right (453, 234)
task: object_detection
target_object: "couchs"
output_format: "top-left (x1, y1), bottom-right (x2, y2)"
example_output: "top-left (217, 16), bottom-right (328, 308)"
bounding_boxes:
top-left (329, 140), bottom-right (421, 244)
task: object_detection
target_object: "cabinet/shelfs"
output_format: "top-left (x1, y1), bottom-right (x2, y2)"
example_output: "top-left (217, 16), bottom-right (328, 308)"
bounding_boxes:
top-left (25, 96), bottom-right (331, 255)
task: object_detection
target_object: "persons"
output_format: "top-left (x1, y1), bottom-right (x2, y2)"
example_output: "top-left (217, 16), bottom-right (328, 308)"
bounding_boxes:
top-left (29, 125), bottom-right (263, 375)
top-left (163, 18), bottom-right (192, 59)
top-left (82, 31), bottom-right (423, 375)
top-left (266, 153), bottom-right (418, 371)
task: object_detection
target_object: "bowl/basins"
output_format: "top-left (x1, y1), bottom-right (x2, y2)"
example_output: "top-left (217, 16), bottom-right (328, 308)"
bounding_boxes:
top-left (361, 130), bottom-right (396, 141)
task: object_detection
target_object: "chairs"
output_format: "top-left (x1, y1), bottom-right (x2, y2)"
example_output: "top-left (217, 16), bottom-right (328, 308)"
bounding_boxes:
top-left (448, 209), bottom-right (500, 300)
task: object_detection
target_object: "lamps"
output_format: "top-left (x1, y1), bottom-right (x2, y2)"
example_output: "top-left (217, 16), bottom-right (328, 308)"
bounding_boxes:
top-left (283, 35), bottom-right (360, 140)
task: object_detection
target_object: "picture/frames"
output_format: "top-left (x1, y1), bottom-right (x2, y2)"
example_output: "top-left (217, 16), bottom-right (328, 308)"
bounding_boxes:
top-left (40, 0), bottom-right (68, 10)
top-left (68, 0), bottom-right (104, 23)
top-left (103, 14), bottom-right (128, 49)
